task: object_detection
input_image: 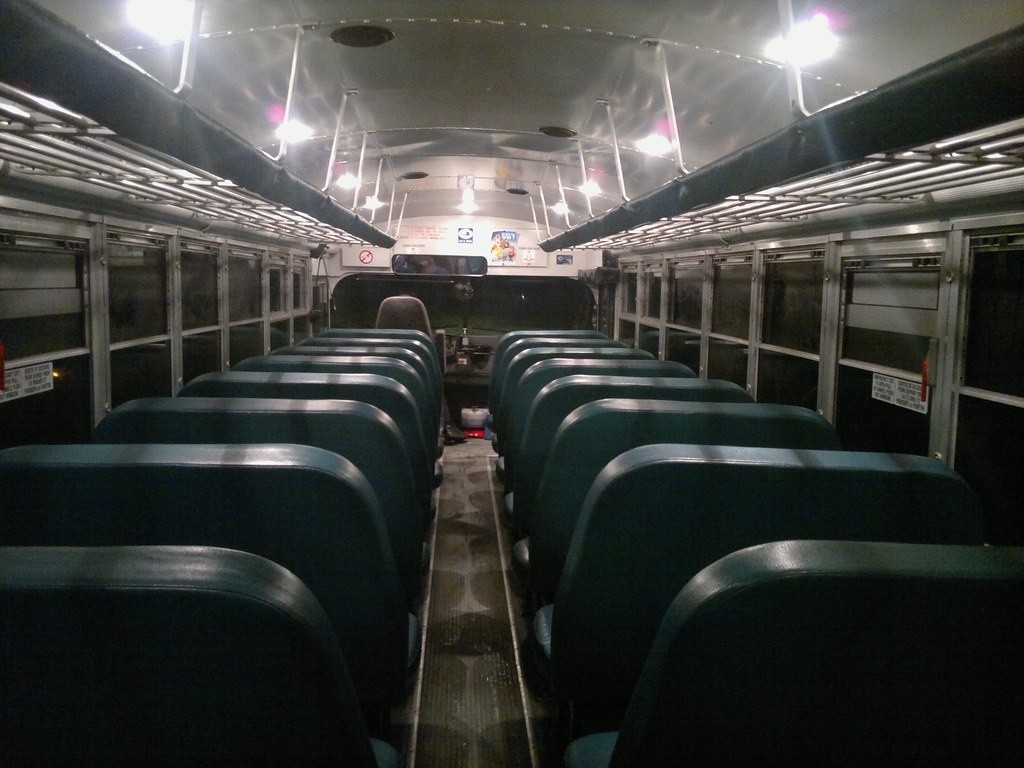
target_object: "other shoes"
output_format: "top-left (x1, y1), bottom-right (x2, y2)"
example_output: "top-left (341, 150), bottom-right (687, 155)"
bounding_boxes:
top-left (445, 424), bottom-right (466, 441)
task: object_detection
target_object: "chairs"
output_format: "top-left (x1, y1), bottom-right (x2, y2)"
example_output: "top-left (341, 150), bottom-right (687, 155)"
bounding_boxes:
top-left (374, 296), bottom-right (434, 339)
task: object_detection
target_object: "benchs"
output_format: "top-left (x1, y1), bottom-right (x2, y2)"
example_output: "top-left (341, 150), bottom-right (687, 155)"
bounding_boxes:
top-left (485, 328), bottom-right (1024, 768)
top-left (0, 323), bottom-right (446, 768)
